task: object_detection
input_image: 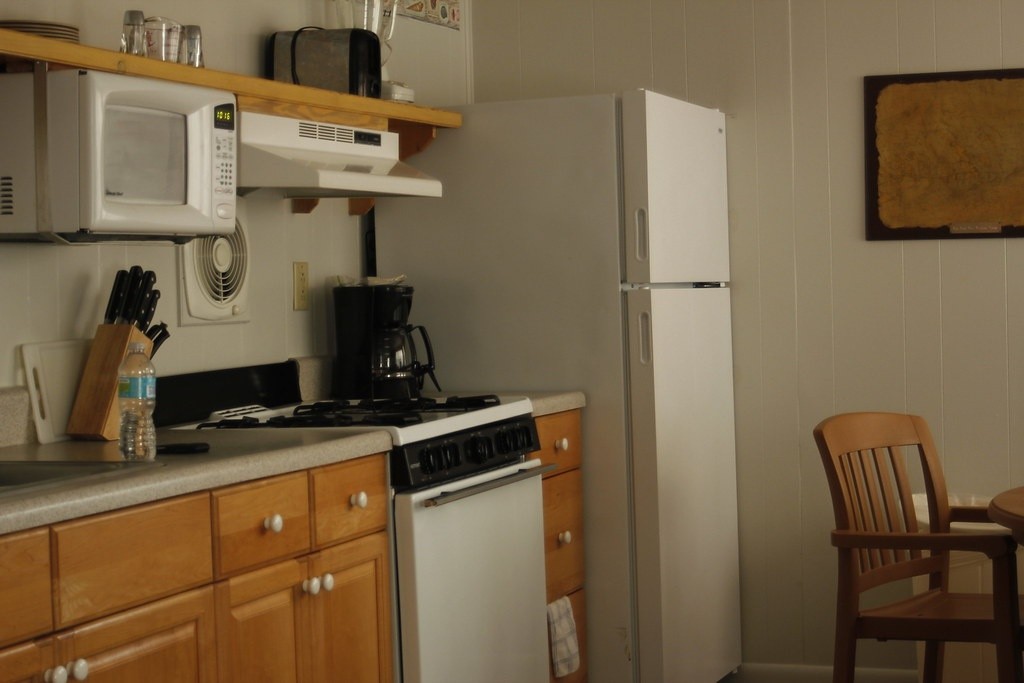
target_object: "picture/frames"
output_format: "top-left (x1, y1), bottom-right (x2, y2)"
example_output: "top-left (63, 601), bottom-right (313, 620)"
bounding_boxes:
top-left (864, 66), bottom-right (1024, 240)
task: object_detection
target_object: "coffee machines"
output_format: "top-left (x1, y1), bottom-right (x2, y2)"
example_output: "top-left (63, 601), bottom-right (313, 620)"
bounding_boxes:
top-left (334, 285), bottom-right (442, 402)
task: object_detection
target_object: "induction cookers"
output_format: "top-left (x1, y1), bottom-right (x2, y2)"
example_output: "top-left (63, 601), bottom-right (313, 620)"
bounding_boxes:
top-left (203, 394), bottom-right (500, 429)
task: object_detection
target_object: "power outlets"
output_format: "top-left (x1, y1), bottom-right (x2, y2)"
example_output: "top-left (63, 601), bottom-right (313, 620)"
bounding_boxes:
top-left (294, 261), bottom-right (309, 310)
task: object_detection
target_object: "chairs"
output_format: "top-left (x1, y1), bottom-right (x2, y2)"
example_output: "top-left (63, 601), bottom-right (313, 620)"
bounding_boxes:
top-left (814, 412), bottom-right (1024, 683)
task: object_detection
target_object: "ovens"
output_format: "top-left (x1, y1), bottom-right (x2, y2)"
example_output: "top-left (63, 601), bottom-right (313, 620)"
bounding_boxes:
top-left (392, 458), bottom-right (551, 683)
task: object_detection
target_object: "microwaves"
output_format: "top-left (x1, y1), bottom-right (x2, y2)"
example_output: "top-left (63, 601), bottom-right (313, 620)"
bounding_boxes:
top-left (0, 70), bottom-right (237, 235)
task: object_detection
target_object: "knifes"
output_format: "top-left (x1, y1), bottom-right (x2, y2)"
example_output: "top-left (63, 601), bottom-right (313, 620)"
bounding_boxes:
top-left (102, 265), bottom-right (171, 364)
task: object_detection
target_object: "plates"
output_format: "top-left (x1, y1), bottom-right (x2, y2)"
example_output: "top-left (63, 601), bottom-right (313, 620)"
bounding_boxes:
top-left (0, 19), bottom-right (80, 50)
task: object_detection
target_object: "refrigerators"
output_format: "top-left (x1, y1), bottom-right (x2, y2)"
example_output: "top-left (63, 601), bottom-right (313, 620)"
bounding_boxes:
top-left (374, 87), bottom-right (743, 683)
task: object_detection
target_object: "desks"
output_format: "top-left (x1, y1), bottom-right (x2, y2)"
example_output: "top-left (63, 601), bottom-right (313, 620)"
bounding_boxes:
top-left (987, 484), bottom-right (1024, 549)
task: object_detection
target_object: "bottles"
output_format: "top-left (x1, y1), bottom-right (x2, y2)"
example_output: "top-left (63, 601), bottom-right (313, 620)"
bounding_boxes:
top-left (120, 342), bottom-right (156, 462)
top-left (120, 10), bottom-right (147, 58)
top-left (145, 15), bottom-right (166, 61)
top-left (165, 18), bottom-right (180, 63)
top-left (185, 25), bottom-right (204, 67)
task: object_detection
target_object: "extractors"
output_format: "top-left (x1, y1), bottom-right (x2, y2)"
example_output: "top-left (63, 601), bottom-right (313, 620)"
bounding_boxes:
top-left (236, 108), bottom-right (442, 211)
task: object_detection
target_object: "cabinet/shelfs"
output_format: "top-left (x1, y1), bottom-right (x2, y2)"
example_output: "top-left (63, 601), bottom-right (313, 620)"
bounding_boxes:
top-left (211, 453), bottom-right (395, 683)
top-left (533, 408), bottom-right (591, 683)
top-left (0, 490), bottom-right (214, 683)
